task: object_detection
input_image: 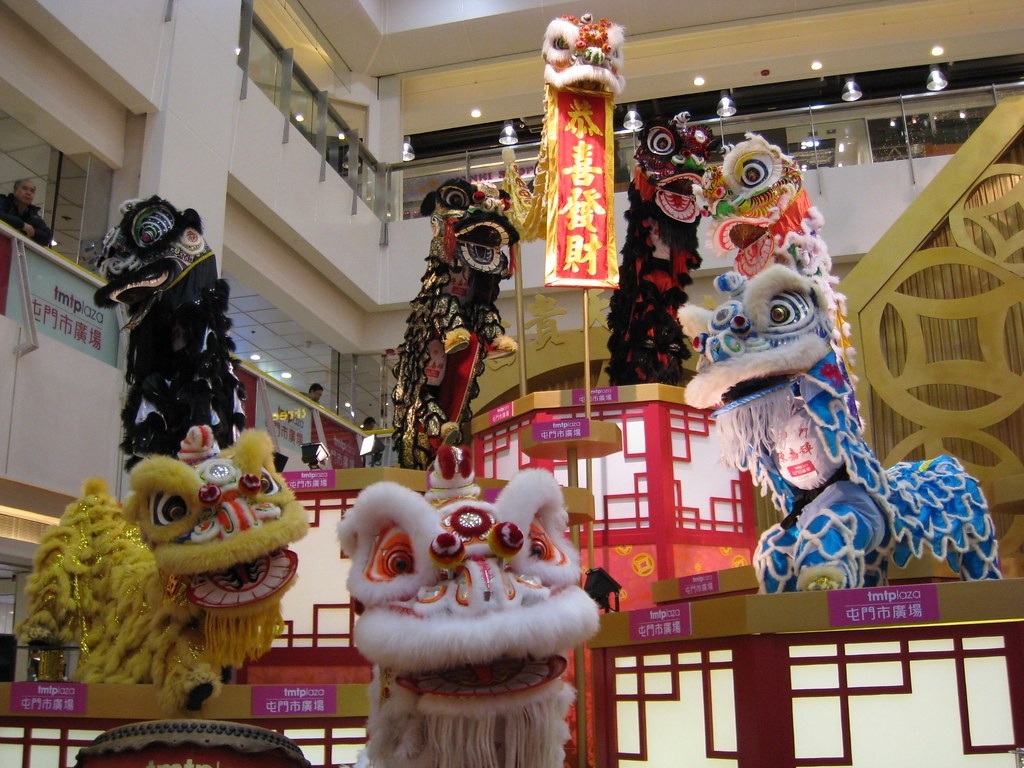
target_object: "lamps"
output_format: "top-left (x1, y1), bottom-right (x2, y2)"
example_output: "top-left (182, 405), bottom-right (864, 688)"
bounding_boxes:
top-left (583, 568), bottom-right (622, 612)
top-left (272, 452), bottom-right (289, 473)
top-left (402, 63), bottom-right (949, 163)
top-left (360, 434), bottom-right (386, 468)
top-left (298, 442), bottom-right (331, 471)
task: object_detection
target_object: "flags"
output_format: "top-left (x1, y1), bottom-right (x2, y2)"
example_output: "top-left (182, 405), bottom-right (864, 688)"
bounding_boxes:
top-left (546, 83), bottom-right (619, 291)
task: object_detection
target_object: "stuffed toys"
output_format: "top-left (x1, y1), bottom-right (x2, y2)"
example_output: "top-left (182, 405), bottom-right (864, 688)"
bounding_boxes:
top-left (338, 444), bottom-right (599, 768)
top-left (678, 262), bottom-right (1004, 593)
top-left (14, 425), bottom-right (310, 716)
top-left (691, 131), bottom-right (863, 422)
top-left (93, 194), bottom-right (246, 468)
top-left (604, 117), bottom-right (712, 386)
top-left (504, 12), bottom-right (626, 243)
top-left (387, 176), bottom-right (521, 469)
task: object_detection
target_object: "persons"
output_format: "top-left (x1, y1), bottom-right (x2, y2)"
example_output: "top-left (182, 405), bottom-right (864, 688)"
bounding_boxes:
top-left (362, 417), bottom-right (375, 431)
top-left (0, 179), bottom-right (50, 247)
top-left (308, 383), bottom-right (323, 402)
